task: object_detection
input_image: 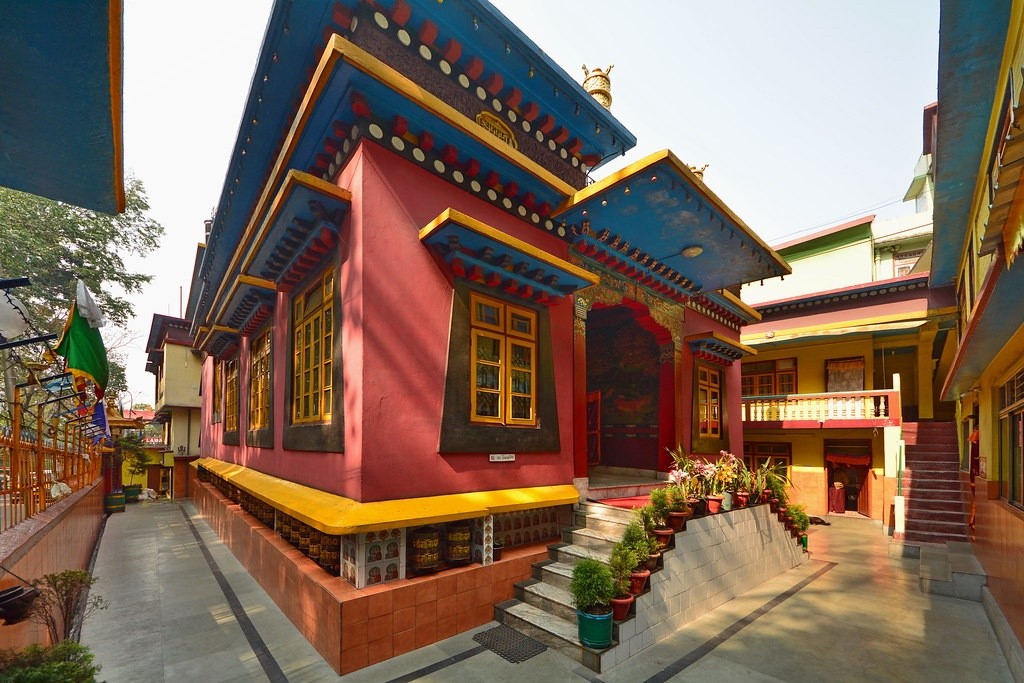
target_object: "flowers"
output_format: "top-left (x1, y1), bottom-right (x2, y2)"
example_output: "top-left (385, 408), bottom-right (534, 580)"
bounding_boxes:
top-left (662, 442), bottom-right (751, 497)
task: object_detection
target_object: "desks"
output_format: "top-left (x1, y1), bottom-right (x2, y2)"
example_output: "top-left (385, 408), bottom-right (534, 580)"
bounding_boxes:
top-left (828, 487), bottom-right (845, 513)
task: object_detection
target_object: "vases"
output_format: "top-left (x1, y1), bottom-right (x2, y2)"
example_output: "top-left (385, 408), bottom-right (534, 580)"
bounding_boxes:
top-left (685, 491), bottom-right (748, 520)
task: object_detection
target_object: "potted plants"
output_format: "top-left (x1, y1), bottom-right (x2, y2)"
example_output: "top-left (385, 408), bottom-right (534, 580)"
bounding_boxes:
top-left (104, 432), bottom-right (155, 513)
top-left (494, 536), bottom-right (505, 561)
top-left (569, 484), bottom-right (690, 649)
top-left (750, 456), bottom-right (809, 551)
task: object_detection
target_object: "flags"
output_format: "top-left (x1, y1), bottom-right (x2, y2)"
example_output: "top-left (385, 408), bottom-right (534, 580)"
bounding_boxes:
top-left (41, 279), bottom-right (137, 446)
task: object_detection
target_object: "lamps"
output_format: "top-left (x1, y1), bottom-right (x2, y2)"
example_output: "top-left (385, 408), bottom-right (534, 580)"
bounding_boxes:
top-left (681, 247), bottom-right (703, 258)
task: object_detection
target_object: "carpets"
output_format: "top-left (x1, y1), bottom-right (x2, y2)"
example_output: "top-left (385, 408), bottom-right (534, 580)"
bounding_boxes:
top-left (472, 624), bottom-right (548, 664)
top-left (587, 494), bottom-right (651, 509)
